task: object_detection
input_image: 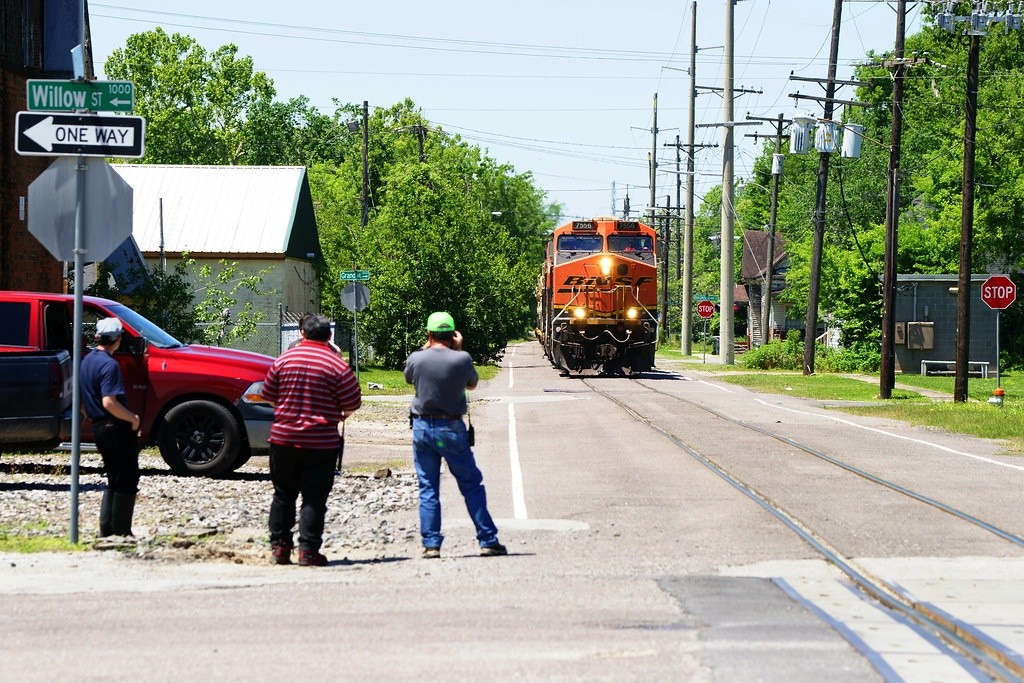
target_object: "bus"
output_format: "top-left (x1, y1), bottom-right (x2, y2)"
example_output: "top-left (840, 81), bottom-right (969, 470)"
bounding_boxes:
top-left (533, 217), bottom-right (668, 377)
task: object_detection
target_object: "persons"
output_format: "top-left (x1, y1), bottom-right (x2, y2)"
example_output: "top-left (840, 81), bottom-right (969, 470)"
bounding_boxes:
top-left (287, 313), bottom-right (343, 358)
top-left (258, 315), bottom-right (362, 567)
top-left (404, 312), bottom-right (507, 558)
top-left (78, 317), bottom-right (141, 536)
top-left (623, 242), bottom-right (651, 260)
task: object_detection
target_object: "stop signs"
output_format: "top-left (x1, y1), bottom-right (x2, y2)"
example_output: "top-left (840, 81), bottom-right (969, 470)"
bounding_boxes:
top-left (981, 276), bottom-right (1016, 309)
top-left (697, 301), bottom-right (714, 318)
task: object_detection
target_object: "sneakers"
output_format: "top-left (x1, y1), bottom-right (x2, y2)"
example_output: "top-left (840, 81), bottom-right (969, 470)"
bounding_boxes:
top-left (480, 543), bottom-right (507, 555)
top-left (269, 540), bottom-right (293, 565)
top-left (421, 547), bottom-right (440, 558)
top-left (299, 543), bottom-right (327, 566)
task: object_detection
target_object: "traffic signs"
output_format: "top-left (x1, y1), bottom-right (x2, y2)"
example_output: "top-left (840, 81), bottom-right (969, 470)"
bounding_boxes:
top-left (14, 112), bottom-right (146, 157)
top-left (25, 79), bottom-right (135, 112)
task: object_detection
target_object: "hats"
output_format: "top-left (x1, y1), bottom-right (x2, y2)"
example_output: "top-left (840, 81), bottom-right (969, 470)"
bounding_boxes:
top-left (96, 318), bottom-right (124, 341)
top-left (427, 312), bottom-right (455, 332)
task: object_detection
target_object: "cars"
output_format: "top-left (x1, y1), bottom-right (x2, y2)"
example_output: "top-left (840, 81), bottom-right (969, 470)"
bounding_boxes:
top-left (0, 289), bottom-right (280, 478)
